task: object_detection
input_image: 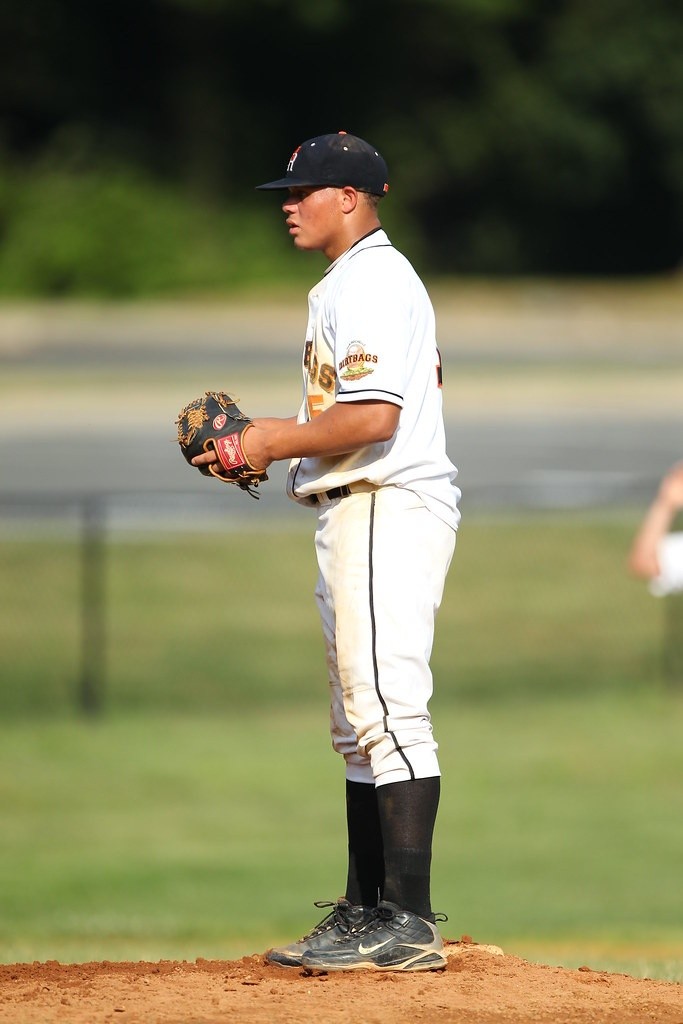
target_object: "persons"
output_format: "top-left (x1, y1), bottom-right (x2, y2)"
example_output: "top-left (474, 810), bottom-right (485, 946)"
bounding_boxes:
top-left (627, 464), bottom-right (683, 600)
top-left (173, 129), bottom-right (461, 973)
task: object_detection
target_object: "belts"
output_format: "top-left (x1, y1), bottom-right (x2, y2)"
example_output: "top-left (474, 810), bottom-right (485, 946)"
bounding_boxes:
top-left (306, 485), bottom-right (348, 504)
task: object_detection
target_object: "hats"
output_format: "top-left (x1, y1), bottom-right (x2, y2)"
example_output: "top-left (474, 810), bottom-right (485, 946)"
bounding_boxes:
top-left (255, 130), bottom-right (389, 196)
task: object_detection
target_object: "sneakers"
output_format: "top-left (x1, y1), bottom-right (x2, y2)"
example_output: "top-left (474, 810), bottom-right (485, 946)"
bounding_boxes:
top-left (265, 894), bottom-right (389, 970)
top-left (300, 904), bottom-right (448, 971)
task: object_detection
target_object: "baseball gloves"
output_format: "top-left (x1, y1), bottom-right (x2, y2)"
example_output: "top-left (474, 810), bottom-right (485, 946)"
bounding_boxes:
top-left (175, 391), bottom-right (270, 502)
